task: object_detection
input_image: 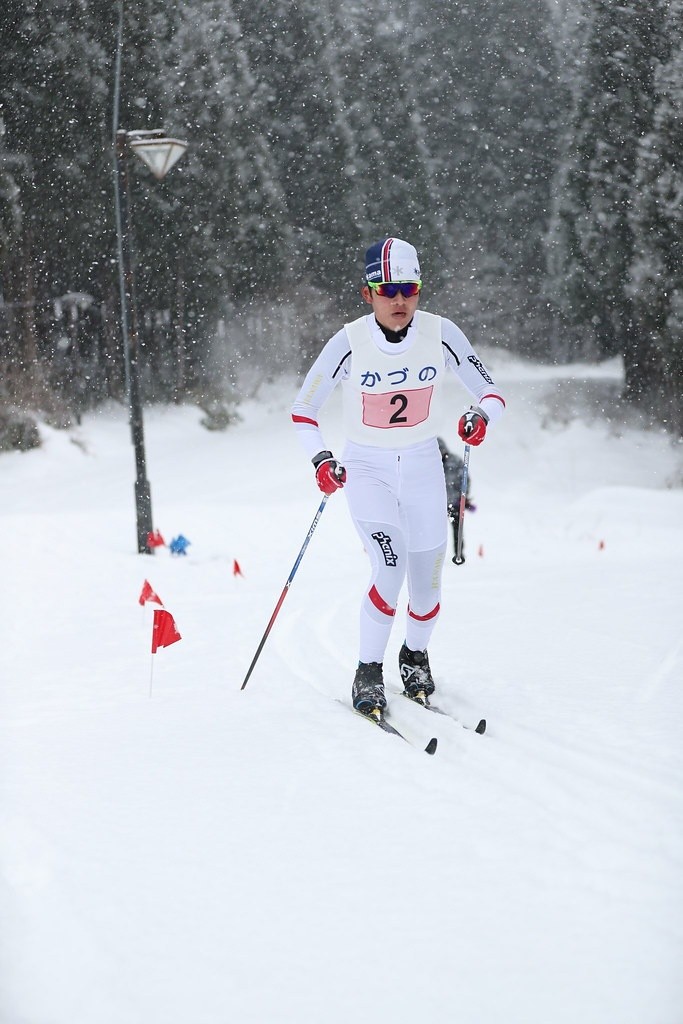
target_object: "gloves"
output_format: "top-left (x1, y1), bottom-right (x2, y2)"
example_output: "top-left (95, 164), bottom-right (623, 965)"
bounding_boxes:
top-left (457, 405), bottom-right (490, 446)
top-left (310, 451), bottom-right (347, 493)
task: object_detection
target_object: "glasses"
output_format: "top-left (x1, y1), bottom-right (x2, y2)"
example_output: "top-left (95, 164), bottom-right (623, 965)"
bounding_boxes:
top-left (367, 280), bottom-right (423, 298)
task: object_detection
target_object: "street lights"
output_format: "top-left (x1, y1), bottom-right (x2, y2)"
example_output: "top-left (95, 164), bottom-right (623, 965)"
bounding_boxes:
top-left (122, 123), bottom-right (193, 554)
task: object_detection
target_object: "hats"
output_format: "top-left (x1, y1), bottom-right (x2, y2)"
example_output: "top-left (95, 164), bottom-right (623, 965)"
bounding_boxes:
top-left (361, 237), bottom-right (421, 282)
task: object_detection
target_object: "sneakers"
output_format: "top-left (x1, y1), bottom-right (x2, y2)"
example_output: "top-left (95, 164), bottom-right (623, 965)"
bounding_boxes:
top-left (351, 660), bottom-right (387, 726)
top-left (398, 638), bottom-right (436, 710)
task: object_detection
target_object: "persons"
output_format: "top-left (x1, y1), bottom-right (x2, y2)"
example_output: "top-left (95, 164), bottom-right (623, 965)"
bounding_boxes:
top-left (437, 436), bottom-right (469, 564)
top-left (289, 237), bottom-right (506, 718)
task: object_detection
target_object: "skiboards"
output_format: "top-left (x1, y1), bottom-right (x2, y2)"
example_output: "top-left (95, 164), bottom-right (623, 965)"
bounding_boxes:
top-left (351, 679), bottom-right (488, 757)
